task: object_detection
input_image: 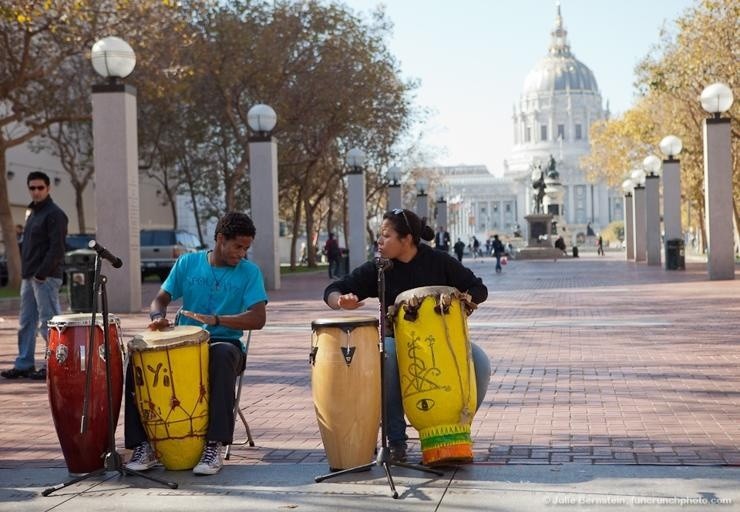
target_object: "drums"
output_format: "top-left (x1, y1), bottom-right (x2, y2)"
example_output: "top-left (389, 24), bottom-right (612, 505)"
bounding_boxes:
top-left (385, 286), bottom-right (477, 467)
top-left (127, 326), bottom-right (210, 471)
top-left (309, 314), bottom-right (382, 473)
top-left (46, 313), bottom-right (125, 477)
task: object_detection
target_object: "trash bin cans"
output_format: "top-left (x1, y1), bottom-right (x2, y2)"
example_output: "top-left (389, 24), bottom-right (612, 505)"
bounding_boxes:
top-left (64, 248), bottom-right (99, 313)
top-left (666, 238), bottom-right (685, 270)
top-left (340, 248), bottom-right (349, 274)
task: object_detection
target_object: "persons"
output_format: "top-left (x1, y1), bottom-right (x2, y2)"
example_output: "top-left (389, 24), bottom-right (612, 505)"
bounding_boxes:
top-left (148, 210), bottom-right (269, 476)
top-left (555, 235), bottom-right (566, 253)
top-left (0, 170), bottom-right (69, 381)
top-left (324, 233), bottom-right (341, 279)
top-left (597, 236), bottom-right (604, 256)
top-left (321, 209), bottom-right (492, 463)
top-left (433, 223), bottom-right (517, 275)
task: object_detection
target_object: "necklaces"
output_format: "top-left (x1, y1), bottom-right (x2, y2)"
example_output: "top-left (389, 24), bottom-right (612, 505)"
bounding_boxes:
top-left (208, 260), bottom-right (232, 289)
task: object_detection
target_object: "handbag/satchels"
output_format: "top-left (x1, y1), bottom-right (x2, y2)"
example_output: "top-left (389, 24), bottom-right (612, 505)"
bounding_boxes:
top-left (500, 255), bottom-right (508, 265)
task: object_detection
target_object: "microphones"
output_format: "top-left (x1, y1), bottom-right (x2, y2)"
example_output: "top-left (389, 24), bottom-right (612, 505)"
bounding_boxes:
top-left (89, 239), bottom-right (123, 268)
top-left (374, 257), bottom-right (393, 271)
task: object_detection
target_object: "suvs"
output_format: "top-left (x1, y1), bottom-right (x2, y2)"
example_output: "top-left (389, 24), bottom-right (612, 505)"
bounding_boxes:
top-left (140, 227), bottom-right (208, 285)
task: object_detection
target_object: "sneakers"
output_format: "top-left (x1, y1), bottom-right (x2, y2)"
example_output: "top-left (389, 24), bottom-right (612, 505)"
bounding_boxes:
top-left (1, 364), bottom-right (46, 380)
top-left (125, 441), bottom-right (159, 471)
top-left (387, 441), bottom-right (406, 460)
top-left (192, 440), bottom-right (225, 475)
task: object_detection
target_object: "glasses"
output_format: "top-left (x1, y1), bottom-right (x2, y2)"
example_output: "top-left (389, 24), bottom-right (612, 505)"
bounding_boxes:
top-left (392, 208), bottom-right (413, 232)
top-left (27, 184), bottom-right (48, 191)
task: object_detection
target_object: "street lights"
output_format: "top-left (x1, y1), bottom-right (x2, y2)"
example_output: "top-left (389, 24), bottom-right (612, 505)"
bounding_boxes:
top-left (244, 100), bottom-right (284, 295)
top-left (699, 78), bottom-right (738, 280)
top-left (345, 145), bottom-right (449, 277)
top-left (622, 132), bottom-right (685, 266)
top-left (85, 34), bottom-right (147, 313)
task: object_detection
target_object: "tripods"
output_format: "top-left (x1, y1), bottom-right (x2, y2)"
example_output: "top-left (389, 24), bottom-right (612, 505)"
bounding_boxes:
top-left (42, 255), bottom-right (178, 497)
top-left (314, 269), bottom-right (444, 499)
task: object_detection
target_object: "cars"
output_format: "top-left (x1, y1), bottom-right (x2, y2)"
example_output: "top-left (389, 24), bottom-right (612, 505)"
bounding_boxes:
top-left (64, 231), bottom-right (98, 251)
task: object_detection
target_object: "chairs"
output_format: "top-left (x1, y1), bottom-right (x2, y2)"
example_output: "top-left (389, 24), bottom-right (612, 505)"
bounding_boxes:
top-left (224, 330), bottom-right (255, 459)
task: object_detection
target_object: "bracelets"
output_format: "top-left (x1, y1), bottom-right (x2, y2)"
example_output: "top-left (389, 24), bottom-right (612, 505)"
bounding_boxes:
top-left (213, 314), bottom-right (219, 327)
top-left (149, 311), bottom-right (166, 320)
